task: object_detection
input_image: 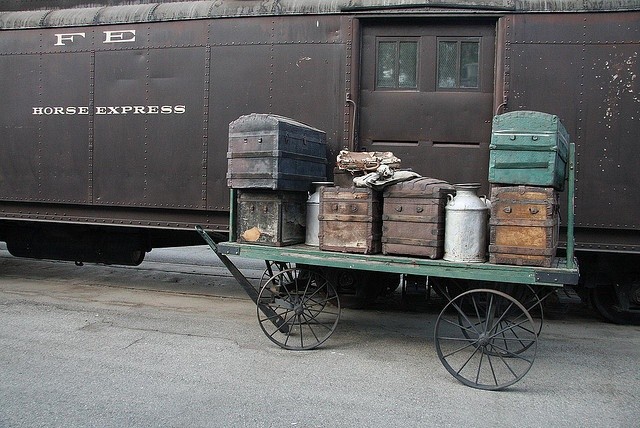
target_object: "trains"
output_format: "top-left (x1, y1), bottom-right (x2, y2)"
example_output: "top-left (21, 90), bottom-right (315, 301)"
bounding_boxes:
top-left (0, 0), bottom-right (639, 325)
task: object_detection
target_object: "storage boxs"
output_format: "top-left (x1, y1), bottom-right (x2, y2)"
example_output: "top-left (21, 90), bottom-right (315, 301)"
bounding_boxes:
top-left (380, 176), bottom-right (455, 260)
top-left (487, 110), bottom-right (570, 187)
top-left (488, 186), bottom-right (560, 267)
top-left (235, 192), bottom-right (307, 246)
top-left (227, 112), bottom-right (329, 193)
top-left (318, 186), bottom-right (376, 255)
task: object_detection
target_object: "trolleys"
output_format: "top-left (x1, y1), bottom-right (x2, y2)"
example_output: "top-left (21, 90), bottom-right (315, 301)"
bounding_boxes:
top-left (195, 143), bottom-right (579, 391)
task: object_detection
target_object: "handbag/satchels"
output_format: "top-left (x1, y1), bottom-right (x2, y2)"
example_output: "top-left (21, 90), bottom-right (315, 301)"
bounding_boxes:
top-left (351, 166), bottom-right (422, 191)
top-left (337, 150), bottom-right (402, 176)
